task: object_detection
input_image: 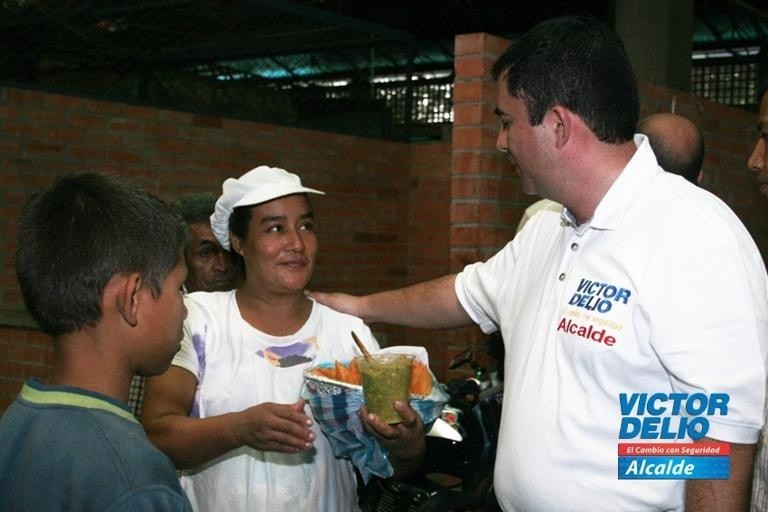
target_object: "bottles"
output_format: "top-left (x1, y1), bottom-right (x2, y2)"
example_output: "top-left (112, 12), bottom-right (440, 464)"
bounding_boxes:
top-left (473, 365), bottom-right (483, 385)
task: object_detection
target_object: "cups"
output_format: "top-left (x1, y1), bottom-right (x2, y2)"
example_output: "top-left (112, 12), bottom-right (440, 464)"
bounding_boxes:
top-left (356, 354), bottom-right (416, 426)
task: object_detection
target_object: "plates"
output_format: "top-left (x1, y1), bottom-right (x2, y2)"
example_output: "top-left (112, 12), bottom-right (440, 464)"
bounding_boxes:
top-left (302, 368), bottom-right (425, 404)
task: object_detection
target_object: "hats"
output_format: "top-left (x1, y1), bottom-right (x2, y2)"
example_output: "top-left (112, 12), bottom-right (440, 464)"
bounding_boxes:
top-left (208, 165), bottom-right (325, 252)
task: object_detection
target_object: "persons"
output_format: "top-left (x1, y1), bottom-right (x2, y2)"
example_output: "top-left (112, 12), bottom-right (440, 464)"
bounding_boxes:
top-left (142, 164), bottom-right (431, 511)
top-left (627, 106), bottom-right (706, 190)
top-left (126, 188), bottom-right (245, 431)
top-left (743, 88), bottom-right (767, 511)
top-left (300, 10), bottom-right (767, 511)
top-left (0, 170), bottom-right (194, 511)
